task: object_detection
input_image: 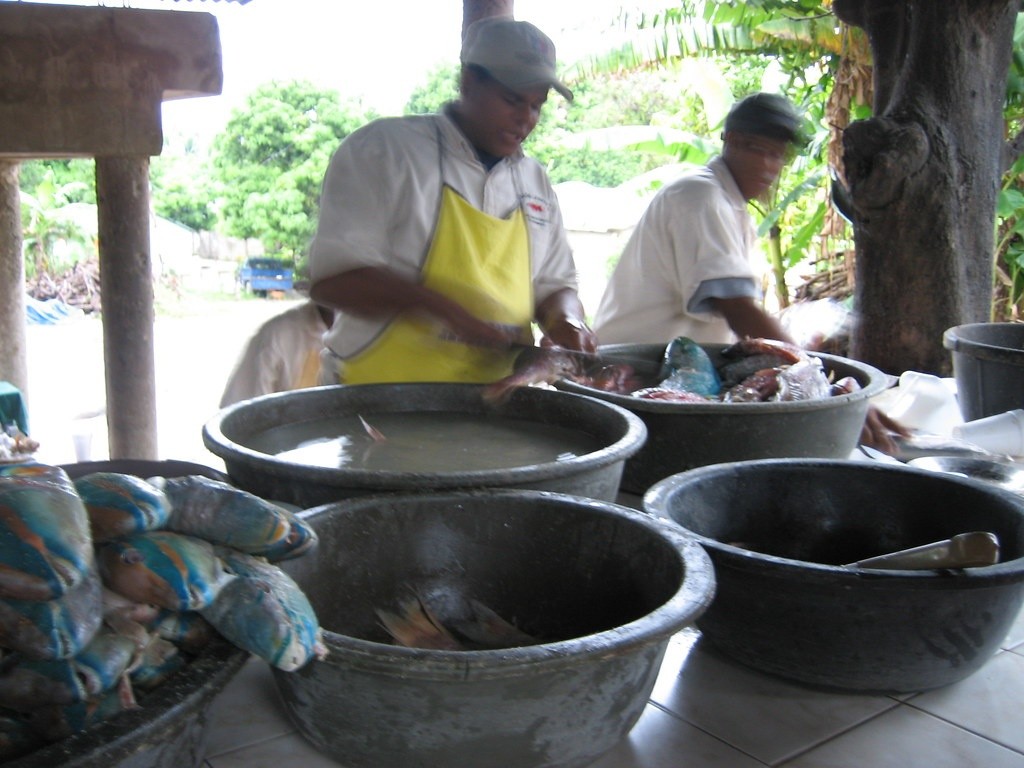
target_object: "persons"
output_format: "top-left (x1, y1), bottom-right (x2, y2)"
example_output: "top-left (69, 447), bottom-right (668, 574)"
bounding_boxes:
top-left (594, 92), bottom-right (912, 454)
top-left (307, 16), bottom-right (598, 389)
top-left (218, 297), bottom-right (335, 411)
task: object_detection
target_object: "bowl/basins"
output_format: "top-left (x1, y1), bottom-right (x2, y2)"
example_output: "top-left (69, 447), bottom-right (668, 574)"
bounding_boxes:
top-left (549, 339), bottom-right (890, 496)
top-left (202, 381), bottom-right (648, 503)
top-left (1, 460), bottom-right (253, 768)
top-left (643, 456), bottom-right (1024, 691)
top-left (268, 489), bottom-right (718, 768)
top-left (943, 323), bottom-right (1024, 423)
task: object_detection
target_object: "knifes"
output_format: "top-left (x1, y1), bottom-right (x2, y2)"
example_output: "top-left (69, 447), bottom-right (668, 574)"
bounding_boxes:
top-left (440, 326), bottom-right (658, 374)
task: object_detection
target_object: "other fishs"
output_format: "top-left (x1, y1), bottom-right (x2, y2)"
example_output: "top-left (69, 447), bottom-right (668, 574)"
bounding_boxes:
top-left (370, 577), bottom-right (541, 652)
top-left (0, 458), bottom-right (332, 768)
top-left (481, 337), bottom-right (863, 403)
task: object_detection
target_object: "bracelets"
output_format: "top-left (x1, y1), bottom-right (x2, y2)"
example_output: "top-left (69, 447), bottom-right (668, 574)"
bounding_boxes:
top-left (563, 318), bottom-right (586, 329)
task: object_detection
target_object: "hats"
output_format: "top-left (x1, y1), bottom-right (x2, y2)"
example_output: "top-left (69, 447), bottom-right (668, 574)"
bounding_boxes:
top-left (462, 17), bottom-right (573, 104)
top-left (726, 92), bottom-right (801, 146)
